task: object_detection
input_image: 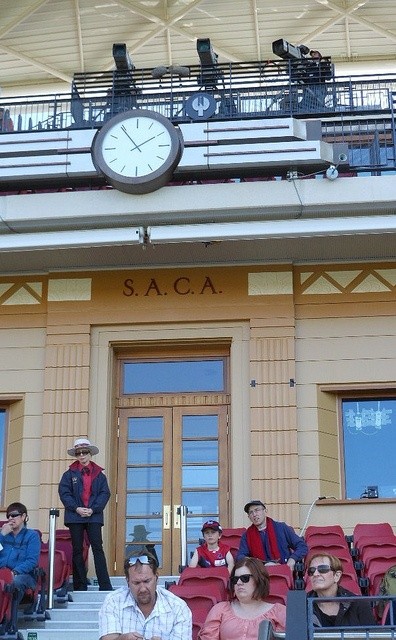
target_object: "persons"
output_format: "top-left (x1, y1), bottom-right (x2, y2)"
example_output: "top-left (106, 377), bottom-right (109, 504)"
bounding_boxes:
top-left (126, 525), bottom-right (159, 567)
top-left (0, 108), bottom-right (15, 134)
top-left (196, 557), bottom-right (286, 640)
top-left (189, 520), bottom-right (235, 575)
top-left (307, 551), bottom-right (377, 640)
top-left (236, 501), bottom-right (309, 572)
top-left (58, 439), bottom-right (114, 591)
top-left (98, 550), bottom-right (193, 640)
top-left (374, 565), bottom-right (396, 640)
top-left (301, 47), bottom-right (334, 110)
top-left (0, 502), bottom-right (42, 635)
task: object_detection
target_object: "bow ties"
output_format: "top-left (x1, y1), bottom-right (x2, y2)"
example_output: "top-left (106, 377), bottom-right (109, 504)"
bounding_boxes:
top-left (81, 467), bottom-right (90, 476)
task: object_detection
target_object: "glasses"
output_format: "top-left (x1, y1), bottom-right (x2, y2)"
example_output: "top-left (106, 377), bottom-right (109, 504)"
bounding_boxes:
top-left (307, 564), bottom-right (331, 576)
top-left (75, 451), bottom-right (90, 457)
top-left (124, 554), bottom-right (152, 568)
top-left (6, 511), bottom-right (21, 519)
top-left (230, 574), bottom-right (252, 584)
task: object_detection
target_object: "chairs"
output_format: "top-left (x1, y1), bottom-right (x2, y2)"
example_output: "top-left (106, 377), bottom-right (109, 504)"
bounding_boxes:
top-left (170, 524), bottom-right (395, 640)
top-left (1, 518), bottom-right (91, 633)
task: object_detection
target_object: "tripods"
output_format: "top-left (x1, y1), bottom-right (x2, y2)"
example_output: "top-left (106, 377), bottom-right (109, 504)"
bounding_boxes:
top-left (265, 59), bottom-right (329, 111)
top-left (91, 71), bottom-right (142, 123)
top-left (173, 66), bottom-right (240, 118)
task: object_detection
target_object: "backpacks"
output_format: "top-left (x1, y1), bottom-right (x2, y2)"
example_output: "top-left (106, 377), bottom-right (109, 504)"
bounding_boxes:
top-left (377, 566), bottom-right (396, 626)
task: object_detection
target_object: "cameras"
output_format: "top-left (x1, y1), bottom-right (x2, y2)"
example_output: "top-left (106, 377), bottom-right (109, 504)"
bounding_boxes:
top-left (196, 38), bottom-right (219, 66)
top-left (272, 38), bottom-right (309, 59)
top-left (112, 43), bottom-right (136, 71)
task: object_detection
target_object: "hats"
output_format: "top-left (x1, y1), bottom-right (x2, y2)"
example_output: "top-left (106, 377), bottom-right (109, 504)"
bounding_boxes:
top-left (201, 520), bottom-right (222, 532)
top-left (244, 500), bottom-right (266, 513)
top-left (129, 525), bottom-right (151, 536)
top-left (67, 439), bottom-right (98, 457)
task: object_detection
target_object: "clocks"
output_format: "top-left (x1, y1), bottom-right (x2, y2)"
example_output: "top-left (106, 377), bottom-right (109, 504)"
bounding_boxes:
top-left (93, 109), bottom-right (181, 194)
